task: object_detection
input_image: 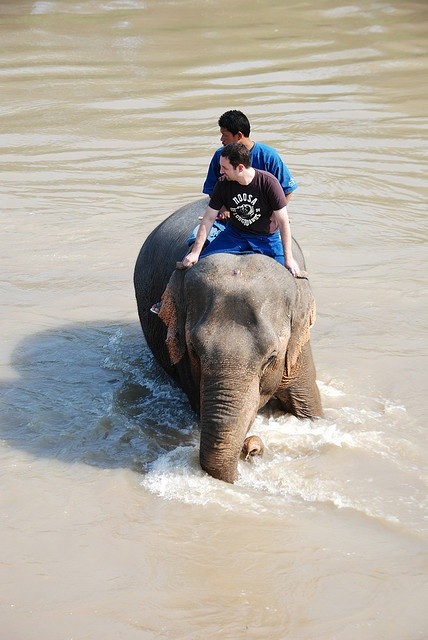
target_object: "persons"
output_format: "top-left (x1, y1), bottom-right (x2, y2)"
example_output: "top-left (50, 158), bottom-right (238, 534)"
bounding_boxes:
top-left (150, 111), bottom-right (298, 314)
top-left (182, 142), bottom-right (301, 277)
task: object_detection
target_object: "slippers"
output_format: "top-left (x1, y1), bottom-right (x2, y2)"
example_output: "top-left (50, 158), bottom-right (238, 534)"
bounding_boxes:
top-left (150, 302), bottom-right (162, 315)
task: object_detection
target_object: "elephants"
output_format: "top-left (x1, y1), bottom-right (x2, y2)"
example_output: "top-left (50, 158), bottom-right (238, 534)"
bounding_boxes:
top-left (134, 195), bottom-right (326, 485)
